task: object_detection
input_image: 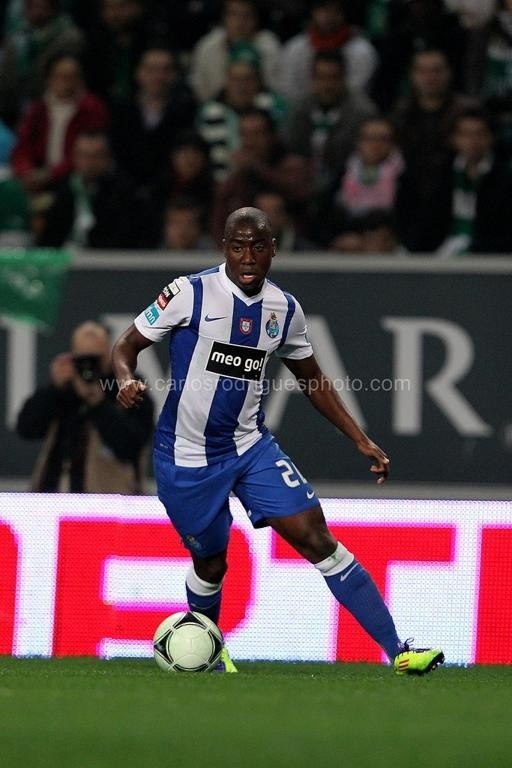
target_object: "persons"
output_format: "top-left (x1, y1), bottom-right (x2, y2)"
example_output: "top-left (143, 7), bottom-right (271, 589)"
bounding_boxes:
top-left (0, 0), bottom-right (511, 263)
top-left (21, 318), bottom-right (156, 495)
top-left (111, 204), bottom-right (451, 678)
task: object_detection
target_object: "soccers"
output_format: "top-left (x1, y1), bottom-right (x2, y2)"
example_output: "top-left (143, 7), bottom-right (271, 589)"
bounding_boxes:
top-left (151, 609), bottom-right (224, 673)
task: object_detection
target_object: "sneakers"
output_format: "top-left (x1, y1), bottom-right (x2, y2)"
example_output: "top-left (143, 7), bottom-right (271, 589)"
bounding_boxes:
top-left (212, 648), bottom-right (237, 674)
top-left (393, 648), bottom-right (445, 678)
